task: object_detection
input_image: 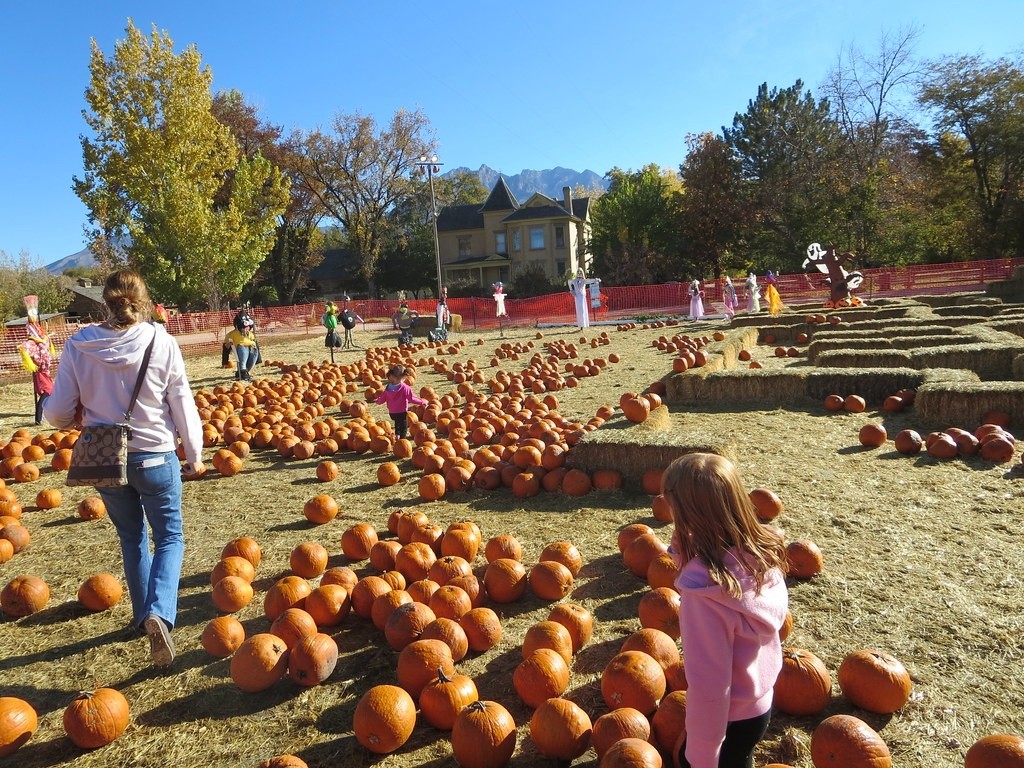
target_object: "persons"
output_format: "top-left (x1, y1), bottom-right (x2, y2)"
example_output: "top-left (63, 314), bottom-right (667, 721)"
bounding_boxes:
top-left (373, 365), bottom-right (427, 439)
top-left (688, 270), bottom-right (785, 323)
top-left (436, 286), bottom-right (451, 328)
top-left (40, 269), bottom-right (204, 666)
top-left (493, 282), bottom-right (507, 317)
top-left (660, 452), bottom-right (790, 768)
top-left (568, 268), bottom-right (602, 328)
top-left (17, 295), bottom-right (56, 425)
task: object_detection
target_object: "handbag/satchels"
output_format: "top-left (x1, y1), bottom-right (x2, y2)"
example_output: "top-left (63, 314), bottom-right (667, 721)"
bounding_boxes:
top-left (65, 425), bottom-right (129, 488)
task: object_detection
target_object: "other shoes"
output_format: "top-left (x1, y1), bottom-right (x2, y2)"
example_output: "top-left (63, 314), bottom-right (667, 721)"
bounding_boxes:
top-left (145, 613), bottom-right (174, 670)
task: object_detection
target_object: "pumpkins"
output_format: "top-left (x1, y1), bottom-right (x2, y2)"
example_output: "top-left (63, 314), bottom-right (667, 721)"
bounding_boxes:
top-left (964, 735), bottom-right (1024, 768)
top-left (0, 316), bottom-right (911, 768)
top-left (824, 389), bottom-right (1015, 463)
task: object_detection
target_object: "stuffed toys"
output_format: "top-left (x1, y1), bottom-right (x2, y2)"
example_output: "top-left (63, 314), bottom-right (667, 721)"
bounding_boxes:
top-left (321, 295), bottom-right (364, 352)
top-left (391, 299), bottom-right (419, 336)
top-left (224, 309), bottom-right (259, 380)
